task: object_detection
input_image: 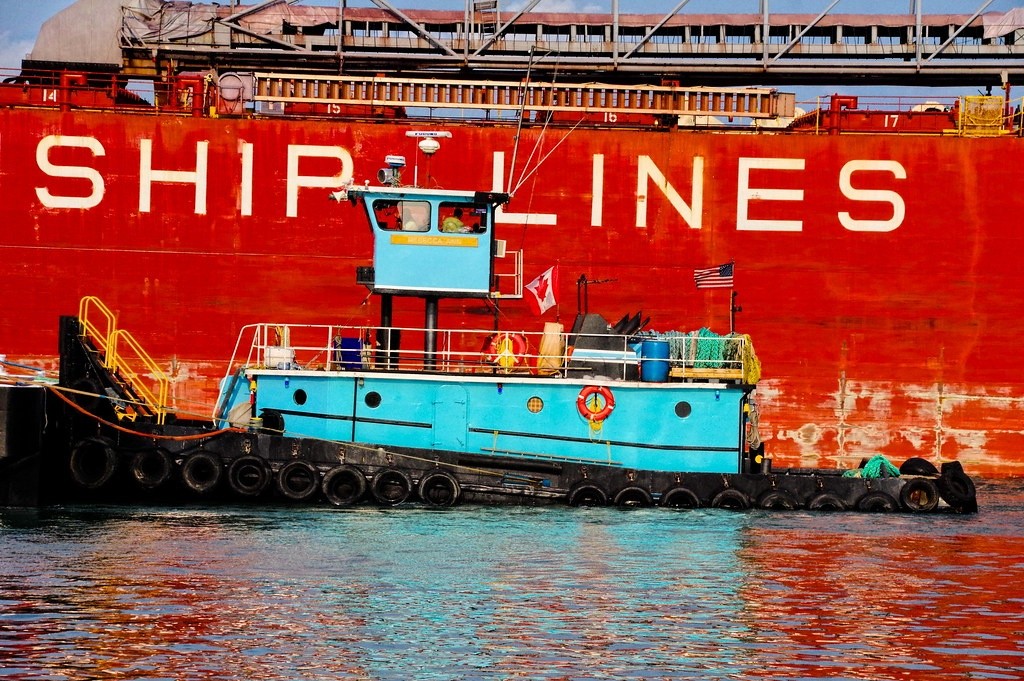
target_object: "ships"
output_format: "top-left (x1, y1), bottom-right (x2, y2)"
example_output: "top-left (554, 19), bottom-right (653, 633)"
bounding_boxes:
top-left (0, 0), bottom-right (1024, 486)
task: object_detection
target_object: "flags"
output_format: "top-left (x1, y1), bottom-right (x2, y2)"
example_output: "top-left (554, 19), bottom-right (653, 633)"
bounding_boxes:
top-left (693, 261), bottom-right (734, 289)
top-left (523, 263), bottom-right (557, 320)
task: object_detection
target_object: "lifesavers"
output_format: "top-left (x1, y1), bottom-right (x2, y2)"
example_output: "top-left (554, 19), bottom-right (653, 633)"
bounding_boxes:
top-left (577, 385), bottom-right (614, 421)
top-left (487, 333), bottom-right (527, 371)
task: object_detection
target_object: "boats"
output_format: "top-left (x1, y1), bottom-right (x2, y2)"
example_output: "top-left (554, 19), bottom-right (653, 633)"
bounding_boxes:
top-left (219, 166), bottom-right (760, 474)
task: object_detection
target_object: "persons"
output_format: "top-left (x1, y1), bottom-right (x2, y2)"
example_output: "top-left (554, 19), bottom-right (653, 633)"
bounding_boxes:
top-left (396, 209), bottom-right (417, 232)
top-left (442, 209), bottom-right (464, 232)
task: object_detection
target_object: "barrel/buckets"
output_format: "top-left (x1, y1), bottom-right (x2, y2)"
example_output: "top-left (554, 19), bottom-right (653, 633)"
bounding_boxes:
top-left (331, 336), bottom-right (369, 370)
top-left (643, 338), bottom-right (668, 382)
